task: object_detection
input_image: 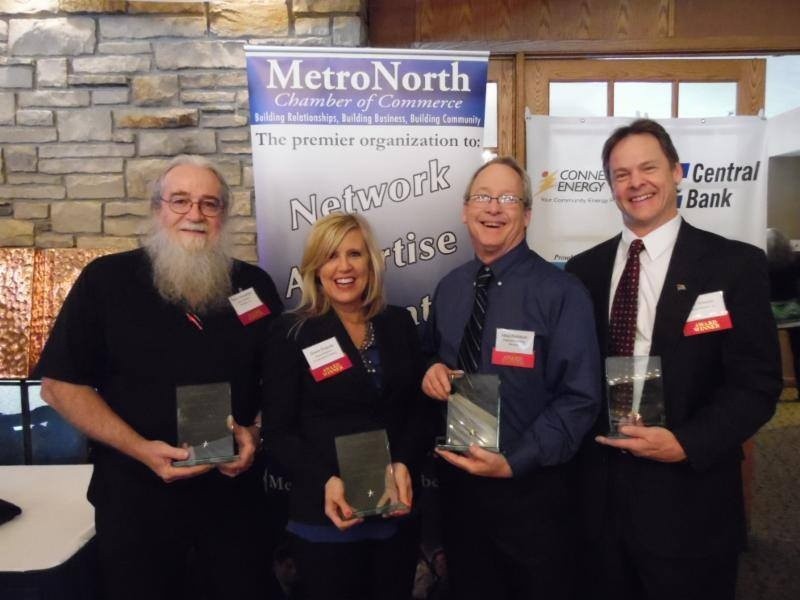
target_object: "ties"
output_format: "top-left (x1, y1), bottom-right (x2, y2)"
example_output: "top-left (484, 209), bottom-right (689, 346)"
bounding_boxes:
top-left (607, 238), bottom-right (645, 421)
top-left (450, 262), bottom-right (494, 396)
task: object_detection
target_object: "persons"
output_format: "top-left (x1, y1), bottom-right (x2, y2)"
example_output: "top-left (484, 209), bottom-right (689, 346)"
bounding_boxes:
top-left (411, 156), bottom-right (600, 600)
top-left (566, 120), bottom-right (784, 600)
top-left (259, 215), bottom-right (429, 600)
top-left (32, 151), bottom-right (286, 600)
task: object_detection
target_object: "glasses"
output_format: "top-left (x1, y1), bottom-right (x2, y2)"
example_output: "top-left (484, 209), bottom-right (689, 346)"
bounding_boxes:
top-left (466, 194), bottom-right (528, 205)
top-left (156, 196), bottom-right (230, 218)
